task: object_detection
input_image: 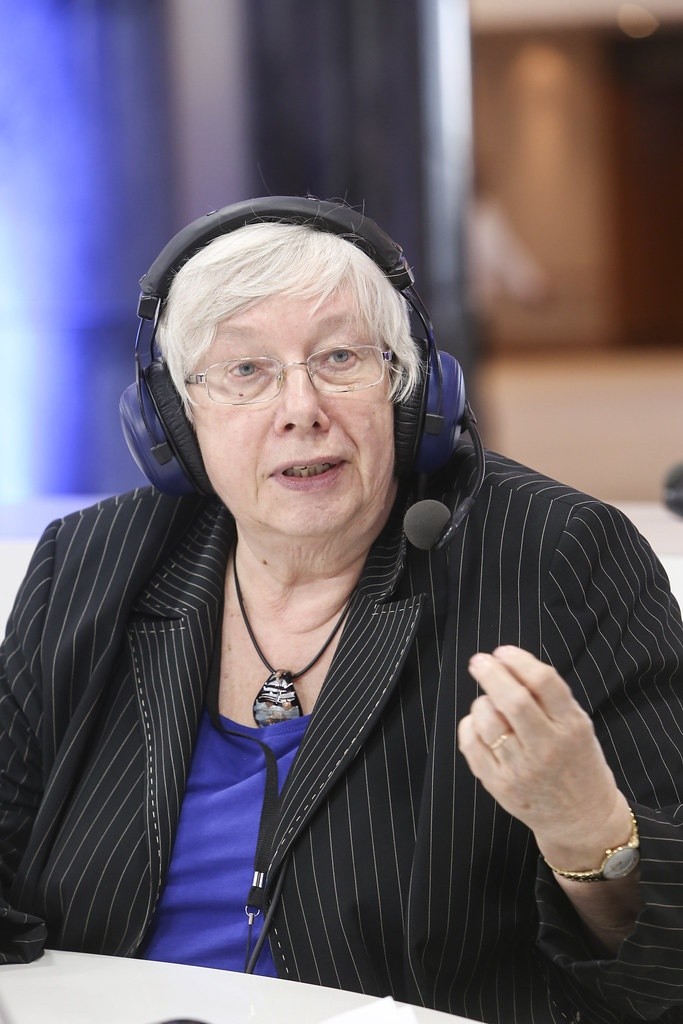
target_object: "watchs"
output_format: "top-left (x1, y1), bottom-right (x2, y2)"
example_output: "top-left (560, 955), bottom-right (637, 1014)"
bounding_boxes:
top-left (544, 807), bottom-right (640, 883)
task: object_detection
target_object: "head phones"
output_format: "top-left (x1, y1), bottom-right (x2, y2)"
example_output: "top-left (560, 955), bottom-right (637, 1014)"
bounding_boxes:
top-left (116, 193), bottom-right (475, 495)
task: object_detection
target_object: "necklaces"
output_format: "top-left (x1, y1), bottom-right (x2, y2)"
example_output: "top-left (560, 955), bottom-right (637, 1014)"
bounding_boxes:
top-left (232, 539), bottom-right (353, 729)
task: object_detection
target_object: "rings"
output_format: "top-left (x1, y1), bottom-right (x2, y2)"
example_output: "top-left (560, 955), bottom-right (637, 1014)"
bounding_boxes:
top-left (490, 734), bottom-right (507, 749)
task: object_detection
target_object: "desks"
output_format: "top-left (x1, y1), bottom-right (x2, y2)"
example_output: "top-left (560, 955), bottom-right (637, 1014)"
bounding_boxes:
top-left (0, 950), bottom-right (484, 1024)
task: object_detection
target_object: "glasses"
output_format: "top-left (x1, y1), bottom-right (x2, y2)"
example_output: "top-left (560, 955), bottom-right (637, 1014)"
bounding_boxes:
top-left (186, 345), bottom-right (395, 405)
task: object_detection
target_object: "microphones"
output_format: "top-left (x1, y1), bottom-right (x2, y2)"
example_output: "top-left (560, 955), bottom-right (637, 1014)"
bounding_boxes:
top-left (402, 421), bottom-right (485, 553)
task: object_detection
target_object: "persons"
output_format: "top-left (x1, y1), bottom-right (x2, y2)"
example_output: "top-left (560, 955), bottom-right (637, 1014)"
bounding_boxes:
top-left (0, 196), bottom-right (683, 1024)
